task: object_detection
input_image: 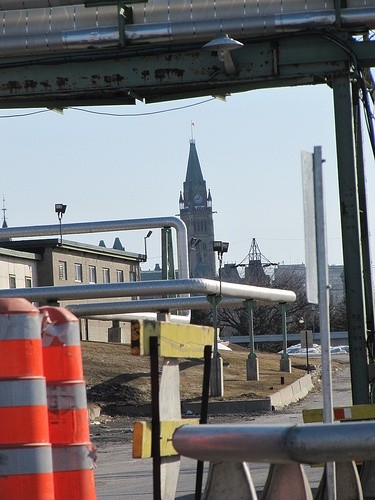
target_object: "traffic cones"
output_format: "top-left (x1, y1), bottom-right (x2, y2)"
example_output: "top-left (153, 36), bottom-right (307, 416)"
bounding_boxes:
top-left (0, 298), bottom-right (100, 499)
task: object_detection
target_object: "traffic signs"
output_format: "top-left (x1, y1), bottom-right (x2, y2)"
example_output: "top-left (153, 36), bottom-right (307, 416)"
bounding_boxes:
top-left (300, 330), bottom-right (313, 348)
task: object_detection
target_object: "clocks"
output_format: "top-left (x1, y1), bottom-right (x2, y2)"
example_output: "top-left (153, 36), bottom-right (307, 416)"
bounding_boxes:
top-left (194, 194), bottom-right (204, 205)
